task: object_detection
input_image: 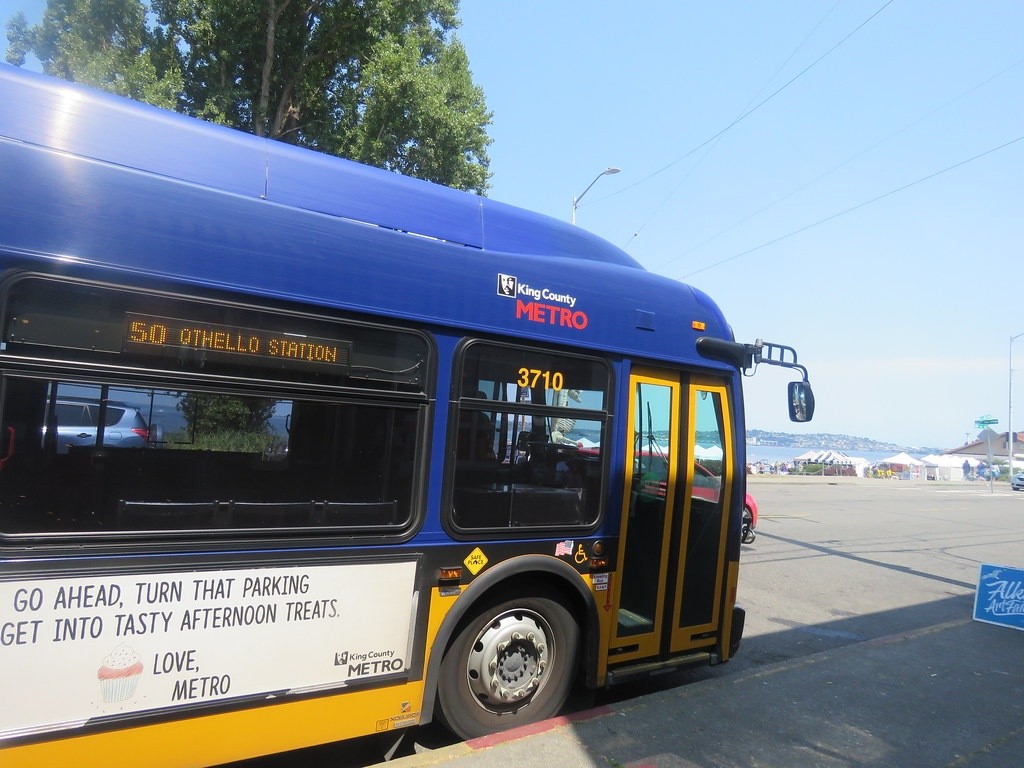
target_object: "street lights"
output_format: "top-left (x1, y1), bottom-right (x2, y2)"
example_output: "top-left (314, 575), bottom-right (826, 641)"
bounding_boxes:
top-left (572, 165), bottom-right (621, 228)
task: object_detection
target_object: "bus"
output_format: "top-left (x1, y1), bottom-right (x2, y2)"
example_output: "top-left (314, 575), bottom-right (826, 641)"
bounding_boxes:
top-left (0, 68), bottom-right (813, 768)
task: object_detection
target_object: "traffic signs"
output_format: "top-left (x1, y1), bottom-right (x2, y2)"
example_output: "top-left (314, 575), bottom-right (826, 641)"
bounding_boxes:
top-left (974, 415), bottom-right (998, 429)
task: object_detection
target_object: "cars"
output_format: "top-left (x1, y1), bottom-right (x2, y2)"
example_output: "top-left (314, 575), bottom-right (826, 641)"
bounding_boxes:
top-left (1011, 468), bottom-right (1024, 490)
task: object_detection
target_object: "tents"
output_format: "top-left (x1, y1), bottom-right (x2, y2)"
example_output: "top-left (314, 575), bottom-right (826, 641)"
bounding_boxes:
top-left (694, 445), bottom-right (724, 461)
top-left (874, 453), bottom-right (925, 480)
top-left (637, 443), bottom-right (670, 457)
top-left (564, 437), bottom-right (600, 451)
top-left (917, 454), bottom-right (987, 480)
top-left (793, 450), bottom-right (856, 477)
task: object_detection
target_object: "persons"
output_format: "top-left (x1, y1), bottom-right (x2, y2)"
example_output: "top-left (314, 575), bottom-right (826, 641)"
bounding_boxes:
top-left (962, 460), bottom-right (970, 479)
top-left (978, 467), bottom-right (984, 477)
top-left (991, 462), bottom-right (999, 481)
top-left (549, 422), bottom-right (583, 448)
top-left (932, 472), bottom-right (935, 480)
top-left (457, 391), bottom-right (496, 462)
top-left (928, 473), bottom-right (931, 480)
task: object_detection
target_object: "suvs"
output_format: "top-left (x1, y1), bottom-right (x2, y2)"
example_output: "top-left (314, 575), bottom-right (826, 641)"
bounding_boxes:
top-left (554, 446), bottom-right (757, 537)
top-left (42, 395), bottom-right (162, 450)
